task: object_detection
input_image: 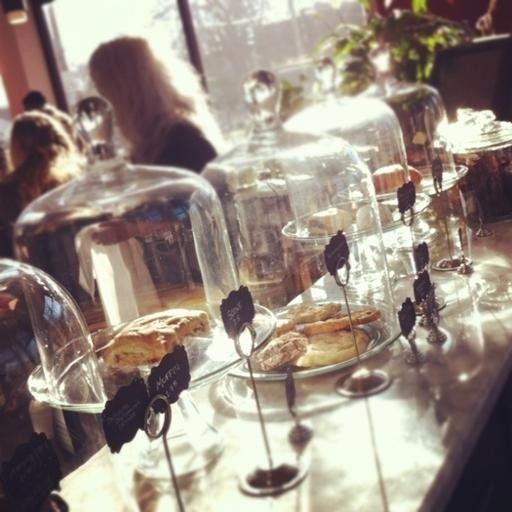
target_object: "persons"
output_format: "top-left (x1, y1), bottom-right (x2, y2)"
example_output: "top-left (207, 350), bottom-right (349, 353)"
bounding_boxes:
top-left (87, 33), bottom-right (226, 291)
top-left (23, 88), bottom-right (74, 137)
top-left (1, 107), bottom-right (99, 303)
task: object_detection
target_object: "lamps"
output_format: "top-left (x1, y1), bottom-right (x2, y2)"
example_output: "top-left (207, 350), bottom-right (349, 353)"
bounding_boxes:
top-left (0, 0), bottom-right (34, 24)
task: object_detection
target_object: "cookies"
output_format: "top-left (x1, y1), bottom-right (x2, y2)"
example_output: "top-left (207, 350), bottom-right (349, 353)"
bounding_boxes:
top-left (252, 300), bottom-right (381, 372)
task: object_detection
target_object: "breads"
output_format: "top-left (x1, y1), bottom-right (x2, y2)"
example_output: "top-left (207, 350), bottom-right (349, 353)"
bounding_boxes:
top-left (94, 308), bottom-right (210, 368)
top-left (356, 203), bottom-right (392, 230)
top-left (307, 206), bottom-right (353, 234)
top-left (372, 163), bottom-right (422, 194)
